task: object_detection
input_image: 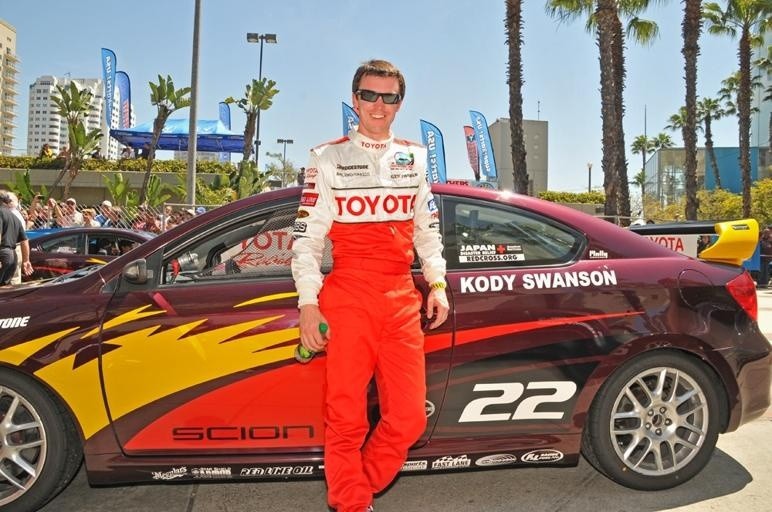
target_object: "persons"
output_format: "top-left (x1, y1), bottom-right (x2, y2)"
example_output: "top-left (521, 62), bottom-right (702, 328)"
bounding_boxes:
top-left (289, 60), bottom-right (450, 512)
top-left (0, 190), bottom-right (207, 286)
top-left (40, 143), bottom-right (52, 158)
top-left (59, 146), bottom-right (68, 156)
top-left (92, 149), bottom-right (102, 158)
top-left (756, 228), bottom-right (772, 289)
top-left (121, 140), bottom-right (155, 159)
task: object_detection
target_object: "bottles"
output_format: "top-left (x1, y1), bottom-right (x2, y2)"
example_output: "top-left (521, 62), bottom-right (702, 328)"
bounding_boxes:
top-left (294, 323), bottom-right (328, 363)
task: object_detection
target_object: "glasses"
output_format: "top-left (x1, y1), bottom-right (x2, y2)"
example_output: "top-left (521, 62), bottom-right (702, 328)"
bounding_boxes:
top-left (357, 90), bottom-right (401, 104)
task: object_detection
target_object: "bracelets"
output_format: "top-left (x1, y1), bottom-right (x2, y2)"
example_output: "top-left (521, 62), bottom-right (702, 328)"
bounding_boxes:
top-left (430, 280), bottom-right (447, 290)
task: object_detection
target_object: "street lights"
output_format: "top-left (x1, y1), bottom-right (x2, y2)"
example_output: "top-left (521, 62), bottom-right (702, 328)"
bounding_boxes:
top-left (277, 139), bottom-right (293, 188)
top-left (247, 33), bottom-right (277, 167)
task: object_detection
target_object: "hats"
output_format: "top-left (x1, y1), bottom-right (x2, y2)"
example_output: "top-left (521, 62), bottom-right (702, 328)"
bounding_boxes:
top-left (102, 200), bottom-right (112, 207)
top-left (196, 207), bottom-right (205, 213)
top-left (66, 198), bottom-right (76, 204)
